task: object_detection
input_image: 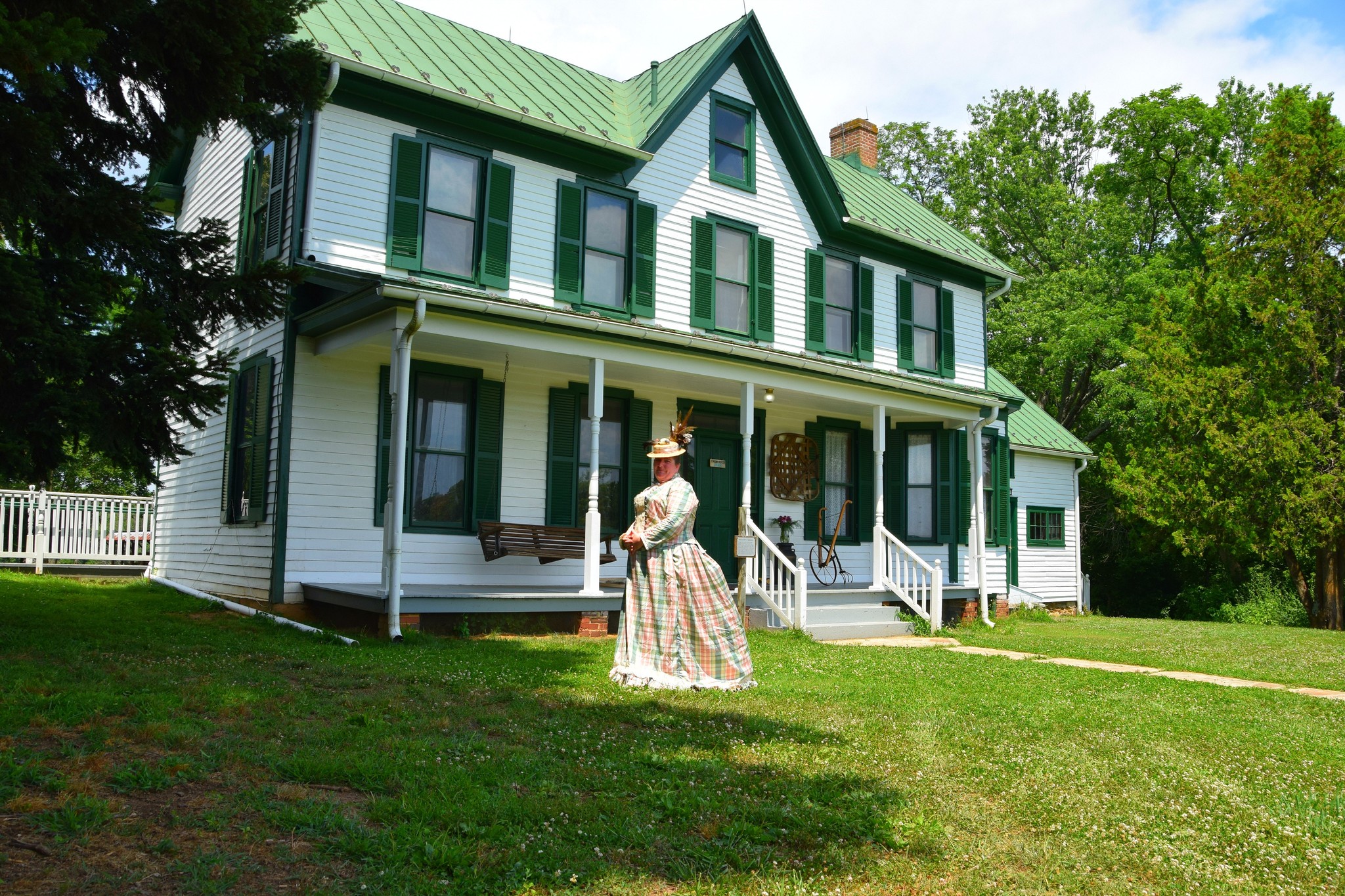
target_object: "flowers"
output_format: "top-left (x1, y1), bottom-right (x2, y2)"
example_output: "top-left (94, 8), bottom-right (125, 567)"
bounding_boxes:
top-left (769, 516), bottom-right (802, 542)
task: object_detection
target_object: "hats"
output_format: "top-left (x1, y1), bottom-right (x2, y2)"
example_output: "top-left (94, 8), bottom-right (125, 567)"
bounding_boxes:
top-left (643, 404), bottom-right (697, 458)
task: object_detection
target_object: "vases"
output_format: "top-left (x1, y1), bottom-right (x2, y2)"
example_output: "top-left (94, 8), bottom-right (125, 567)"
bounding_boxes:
top-left (780, 529), bottom-right (790, 543)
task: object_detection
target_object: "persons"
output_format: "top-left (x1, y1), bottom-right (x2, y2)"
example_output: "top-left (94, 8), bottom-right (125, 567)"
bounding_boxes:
top-left (609, 404), bottom-right (759, 691)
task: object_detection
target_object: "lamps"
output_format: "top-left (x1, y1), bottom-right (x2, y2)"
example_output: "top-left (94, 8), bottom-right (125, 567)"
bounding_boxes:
top-left (764, 388), bottom-right (775, 403)
